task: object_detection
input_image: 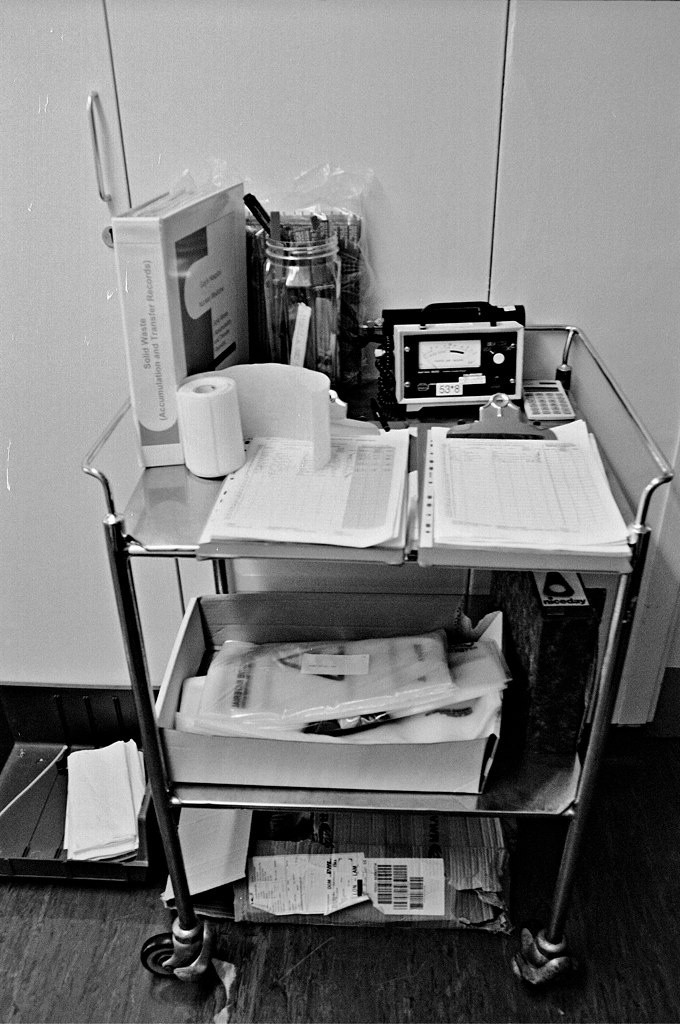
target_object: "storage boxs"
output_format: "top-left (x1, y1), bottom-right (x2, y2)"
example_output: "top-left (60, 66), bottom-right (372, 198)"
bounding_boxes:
top-left (155, 590), bottom-right (505, 796)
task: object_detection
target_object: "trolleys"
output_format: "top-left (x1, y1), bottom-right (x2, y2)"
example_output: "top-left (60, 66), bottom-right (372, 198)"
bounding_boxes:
top-left (82, 319), bottom-right (676, 987)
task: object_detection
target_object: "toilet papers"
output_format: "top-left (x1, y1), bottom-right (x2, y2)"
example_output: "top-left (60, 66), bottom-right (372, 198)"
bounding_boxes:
top-left (175, 363), bottom-right (333, 476)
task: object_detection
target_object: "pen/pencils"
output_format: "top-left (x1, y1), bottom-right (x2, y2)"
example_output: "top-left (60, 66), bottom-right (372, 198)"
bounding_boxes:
top-left (368, 396), bottom-right (390, 432)
top-left (243, 193), bottom-right (342, 387)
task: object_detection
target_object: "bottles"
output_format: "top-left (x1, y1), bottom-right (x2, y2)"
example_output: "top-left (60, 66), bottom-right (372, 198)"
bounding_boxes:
top-left (263, 226), bottom-right (342, 389)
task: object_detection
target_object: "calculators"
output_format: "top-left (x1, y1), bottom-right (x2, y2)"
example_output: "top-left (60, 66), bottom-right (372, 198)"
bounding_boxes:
top-left (522, 377), bottom-right (576, 421)
